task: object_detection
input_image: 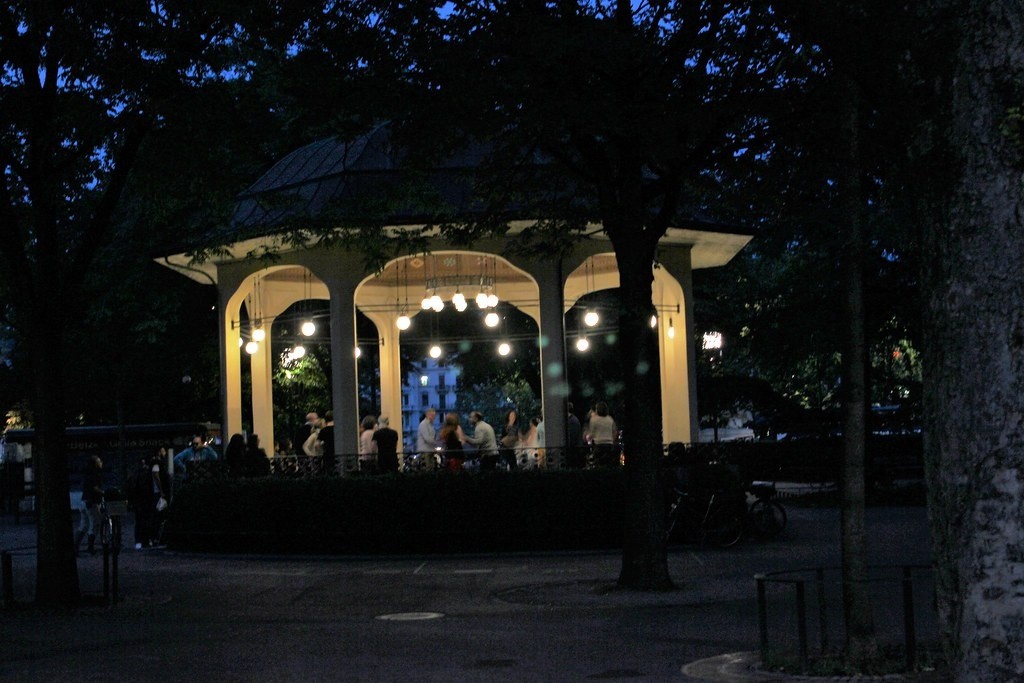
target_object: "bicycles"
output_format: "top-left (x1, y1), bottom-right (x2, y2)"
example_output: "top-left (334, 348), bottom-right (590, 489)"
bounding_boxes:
top-left (660, 478), bottom-right (746, 547)
top-left (748, 466), bottom-right (792, 538)
top-left (98, 487), bottom-right (122, 549)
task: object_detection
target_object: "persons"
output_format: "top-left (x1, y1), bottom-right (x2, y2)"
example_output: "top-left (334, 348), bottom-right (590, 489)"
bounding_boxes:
top-left (71, 396), bottom-right (622, 557)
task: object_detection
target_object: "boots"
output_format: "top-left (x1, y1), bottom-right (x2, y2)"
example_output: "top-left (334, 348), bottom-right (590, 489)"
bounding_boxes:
top-left (74, 530), bottom-right (98, 556)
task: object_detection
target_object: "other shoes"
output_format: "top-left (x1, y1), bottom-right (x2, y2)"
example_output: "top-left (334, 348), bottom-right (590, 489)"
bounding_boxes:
top-left (133, 542), bottom-right (166, 552)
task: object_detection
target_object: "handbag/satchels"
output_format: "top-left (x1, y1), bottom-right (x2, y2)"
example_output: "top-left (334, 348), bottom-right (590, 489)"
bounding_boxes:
top-left (156, 497), bottom-right (167, 511)
